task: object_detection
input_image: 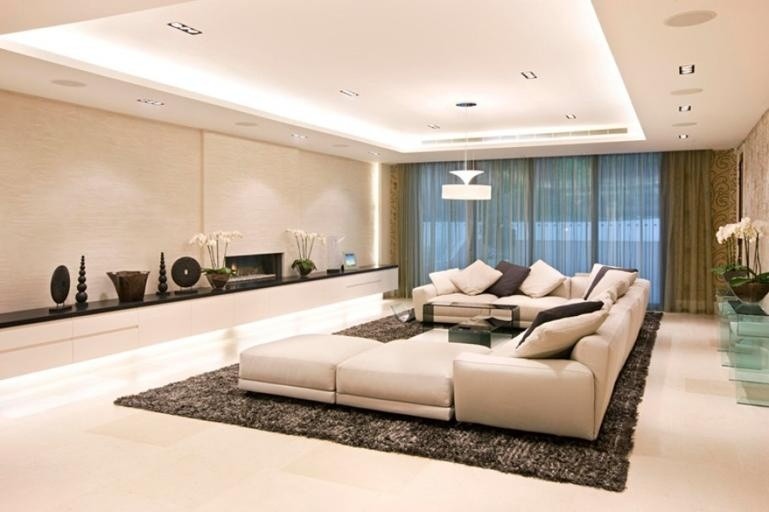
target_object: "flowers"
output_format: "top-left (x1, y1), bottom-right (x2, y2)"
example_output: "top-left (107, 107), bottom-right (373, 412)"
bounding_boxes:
top-left (187, 229), bottom-right (244, 281)
top-left (726, 215), bottom-right (769, 291)
top-left (284, 227), bottom-right (327, 273)
top-left (708, 223), bottom-right (756, 279)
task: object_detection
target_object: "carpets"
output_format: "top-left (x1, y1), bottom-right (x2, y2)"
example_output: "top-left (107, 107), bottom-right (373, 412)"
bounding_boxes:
top-left (111, 310), bottom-right (668, 492)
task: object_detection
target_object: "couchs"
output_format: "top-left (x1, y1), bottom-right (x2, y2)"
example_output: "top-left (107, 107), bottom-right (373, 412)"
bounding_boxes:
top-left (451, 281), bottom-right (651, 448)
top-left (409, 275), bottom-right (651, 328)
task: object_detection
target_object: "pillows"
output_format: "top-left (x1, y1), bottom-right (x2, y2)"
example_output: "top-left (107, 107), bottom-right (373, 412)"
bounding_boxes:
top-left (428, 266), bottom-right (462, 298)
top-left (511, 259), bottom-right (640, 365)
top-left (449, 256), bottom-right (504, 298)
top-left (486, 259), bottom-right (531, 298)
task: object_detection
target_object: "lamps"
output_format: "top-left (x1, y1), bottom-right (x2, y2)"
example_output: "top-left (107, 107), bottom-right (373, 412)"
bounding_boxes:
top-left (441, 134), bottom-right (493, 203)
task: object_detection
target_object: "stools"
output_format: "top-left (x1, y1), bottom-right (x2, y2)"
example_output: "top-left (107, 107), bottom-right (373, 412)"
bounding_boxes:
top-left (335, 339), bottom-right (455, 429)
top-left (235, 329), bottom-right (385, 406)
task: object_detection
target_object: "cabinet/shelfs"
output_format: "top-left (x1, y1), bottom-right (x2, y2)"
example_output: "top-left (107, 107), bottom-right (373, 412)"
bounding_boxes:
top-left (1, 262), bottom-right (400, 395)
top-left (709, 284), bottom-right (738, 354)
top-left (720, 301), bottom-right (769, 408)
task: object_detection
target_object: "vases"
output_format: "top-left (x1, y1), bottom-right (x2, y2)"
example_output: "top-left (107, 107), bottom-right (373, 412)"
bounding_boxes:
top-left (723, 269), bottom-right (747, 284)
top-left (204, 271), bottom-right (234, 291)
top-left (296, 264), bottom-right (313, 277)
top-left (725, 277), bottom-right (768, 305)
